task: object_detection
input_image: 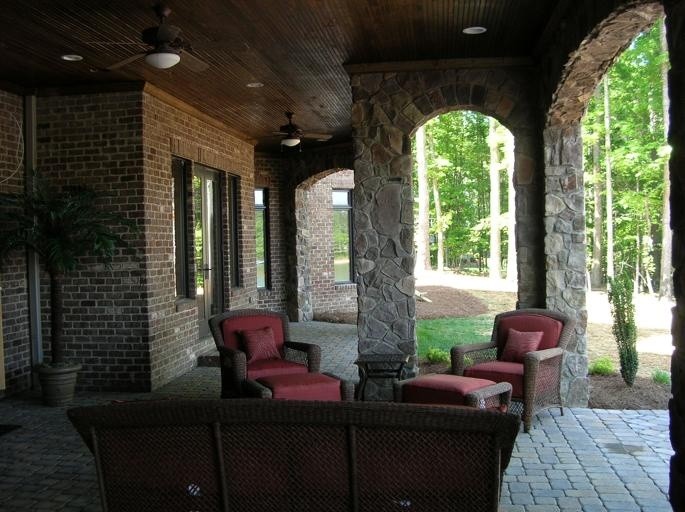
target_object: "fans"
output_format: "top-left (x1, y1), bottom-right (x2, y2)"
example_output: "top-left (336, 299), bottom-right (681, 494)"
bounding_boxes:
top-left (81, 6), bottom-right (249, 72)
top-left (242, 112), bottom-right (333, 152)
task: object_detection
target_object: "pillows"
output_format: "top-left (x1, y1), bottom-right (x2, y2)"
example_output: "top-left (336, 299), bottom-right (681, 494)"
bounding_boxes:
top-left (500, 326), bottom-right (544, 363)
top-left (235, 324), bottom-right (282, 362)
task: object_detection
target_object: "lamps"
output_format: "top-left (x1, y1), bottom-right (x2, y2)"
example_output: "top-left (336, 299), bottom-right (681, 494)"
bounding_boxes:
top-left (145, 49), bottom-right (181, 70)
top-left (280, 137), bottom-right (301, 148)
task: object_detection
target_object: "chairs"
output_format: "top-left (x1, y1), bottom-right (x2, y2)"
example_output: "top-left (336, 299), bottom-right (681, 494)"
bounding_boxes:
top-left (450, 304), bottom-right (575, 432)
top-left (207, 307), bottom-right (346, 403)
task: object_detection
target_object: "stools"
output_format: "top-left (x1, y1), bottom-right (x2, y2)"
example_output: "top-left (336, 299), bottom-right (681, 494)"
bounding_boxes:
top-left (399, 373), bottom-right (513, 413)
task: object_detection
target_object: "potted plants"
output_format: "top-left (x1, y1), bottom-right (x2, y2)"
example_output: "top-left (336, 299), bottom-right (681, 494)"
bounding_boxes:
top-left (0, 169), bottom-right (137, 408)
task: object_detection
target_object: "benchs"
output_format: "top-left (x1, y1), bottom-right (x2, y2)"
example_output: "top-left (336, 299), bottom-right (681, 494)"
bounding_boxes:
top-left (66, 396), bottom-right (523, 509)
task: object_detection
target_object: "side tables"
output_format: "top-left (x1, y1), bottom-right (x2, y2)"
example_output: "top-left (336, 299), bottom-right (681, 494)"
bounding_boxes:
top-left (353, 354), bottom-right (412, 403)
